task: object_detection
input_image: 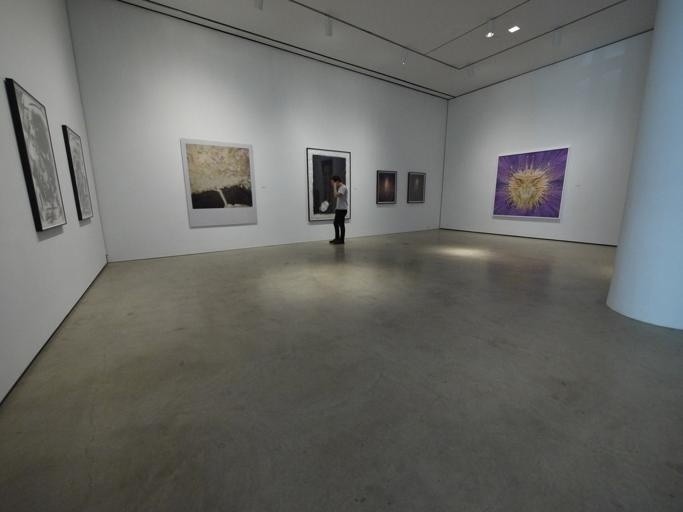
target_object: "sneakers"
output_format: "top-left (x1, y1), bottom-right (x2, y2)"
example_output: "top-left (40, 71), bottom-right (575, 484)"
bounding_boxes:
top-left (329, 240), bottom-right (344, 244)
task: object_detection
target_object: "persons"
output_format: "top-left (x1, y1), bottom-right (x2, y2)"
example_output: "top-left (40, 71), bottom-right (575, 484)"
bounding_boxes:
top-left (328, 175), bottom-right (348, 245)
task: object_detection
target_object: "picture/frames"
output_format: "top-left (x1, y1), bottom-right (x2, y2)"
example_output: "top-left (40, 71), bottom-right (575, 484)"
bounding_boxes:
top-left (406, 172), bottom-right (426, 204)
top-left (305, 147), bottom-right (351, 222)
top-left (490, 145), bottom-right (568, 220)
top-left (375, 170), bottom-right (397, 204)
top-left (3, 77), bottom-right (93, 233)
